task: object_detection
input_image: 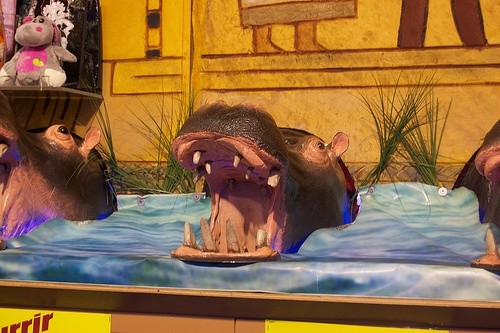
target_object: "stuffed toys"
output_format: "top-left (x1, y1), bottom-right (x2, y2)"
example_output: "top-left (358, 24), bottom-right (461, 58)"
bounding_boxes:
top-left (0, 16), bottom-right (77, 87)
